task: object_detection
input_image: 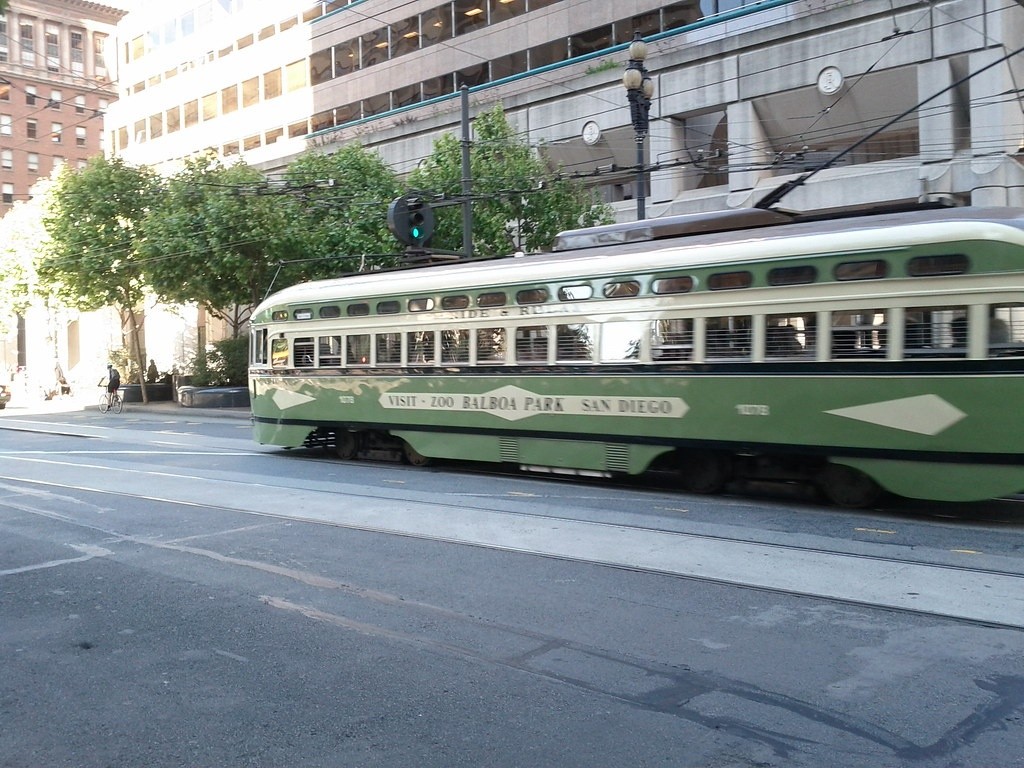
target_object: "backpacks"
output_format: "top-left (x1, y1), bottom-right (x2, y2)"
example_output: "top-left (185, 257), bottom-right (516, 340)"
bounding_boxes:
top-left (111, 369), bottom-right (120, 379)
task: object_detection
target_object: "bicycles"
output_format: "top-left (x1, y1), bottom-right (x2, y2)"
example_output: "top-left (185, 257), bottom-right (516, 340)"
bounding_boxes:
top-left (97, 384), bottom-right (123, 415)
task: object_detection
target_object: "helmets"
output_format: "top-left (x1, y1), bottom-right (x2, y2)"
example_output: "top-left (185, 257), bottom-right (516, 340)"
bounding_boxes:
top-left (107, 364), bottom-right (112, 368)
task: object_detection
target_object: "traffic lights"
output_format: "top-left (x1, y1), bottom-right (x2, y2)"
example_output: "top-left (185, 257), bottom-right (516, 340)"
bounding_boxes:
top-left (386, 193), bottom-right (435, 247)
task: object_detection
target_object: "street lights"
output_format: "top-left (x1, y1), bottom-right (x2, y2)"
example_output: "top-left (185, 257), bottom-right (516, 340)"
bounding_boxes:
top-left (621, 27), bottom-right (657, 221)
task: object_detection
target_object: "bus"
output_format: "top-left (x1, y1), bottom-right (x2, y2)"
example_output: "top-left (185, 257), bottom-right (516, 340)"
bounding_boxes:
top-left (246, 47), bottom-right (1024, 518)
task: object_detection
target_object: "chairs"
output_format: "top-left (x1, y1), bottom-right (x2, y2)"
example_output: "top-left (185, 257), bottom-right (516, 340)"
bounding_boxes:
top-left (533, 337), bottom-right (547, 361)
top-left (718, 328), bottom-right (730, 347)
top-left (878, 322), bottom-right (886, 349)
top-left (577, 335), bottom-right (590, 360)
top-left (516, 339), bottom-right (532, 361)
top-left (319, 343), bottom-right (337, 366)
top-left (559, 332), bottom-right (574, 359)
top-left (455, 346), bottom-right (495, 361)
top-left (766, 321), bottom-right (816, 356)
top-left (744, 318), bottom-right (750, 356)
top-left (388, 337), bottom-right (453, 362)
top-left (951, 316), bottom-right (967, 348)
top-left (337, 341), bottom-right (357, 364)
top-left (734, 320), bottom-right (744, 351)
top-left (833, 330), bottom-right (859, 353)
top-left (906, 319), bottom-right (923, 350)
top-left (861, 322), bottom-right (873, 349)
top-left (990, 318), bottom-right (1009, 344)
top-left (706, 328), bottom-right (716, 356)
top-left (656, 322), bottom-right (693, 362)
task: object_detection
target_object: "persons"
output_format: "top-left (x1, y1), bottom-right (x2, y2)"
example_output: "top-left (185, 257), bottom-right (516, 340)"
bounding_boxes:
top-left (950, 317), bottom-right (966, 348)
top-left (147, 359), bottom-right (160, 383)
top-left (835, 323), bottom-right (887, 358)
top-left (765, 325), bottom-right (802, 357)
top-left (989, 320), bottom-right (1011, 346)
top-left (98, 365), bottom-right (120, 409)
